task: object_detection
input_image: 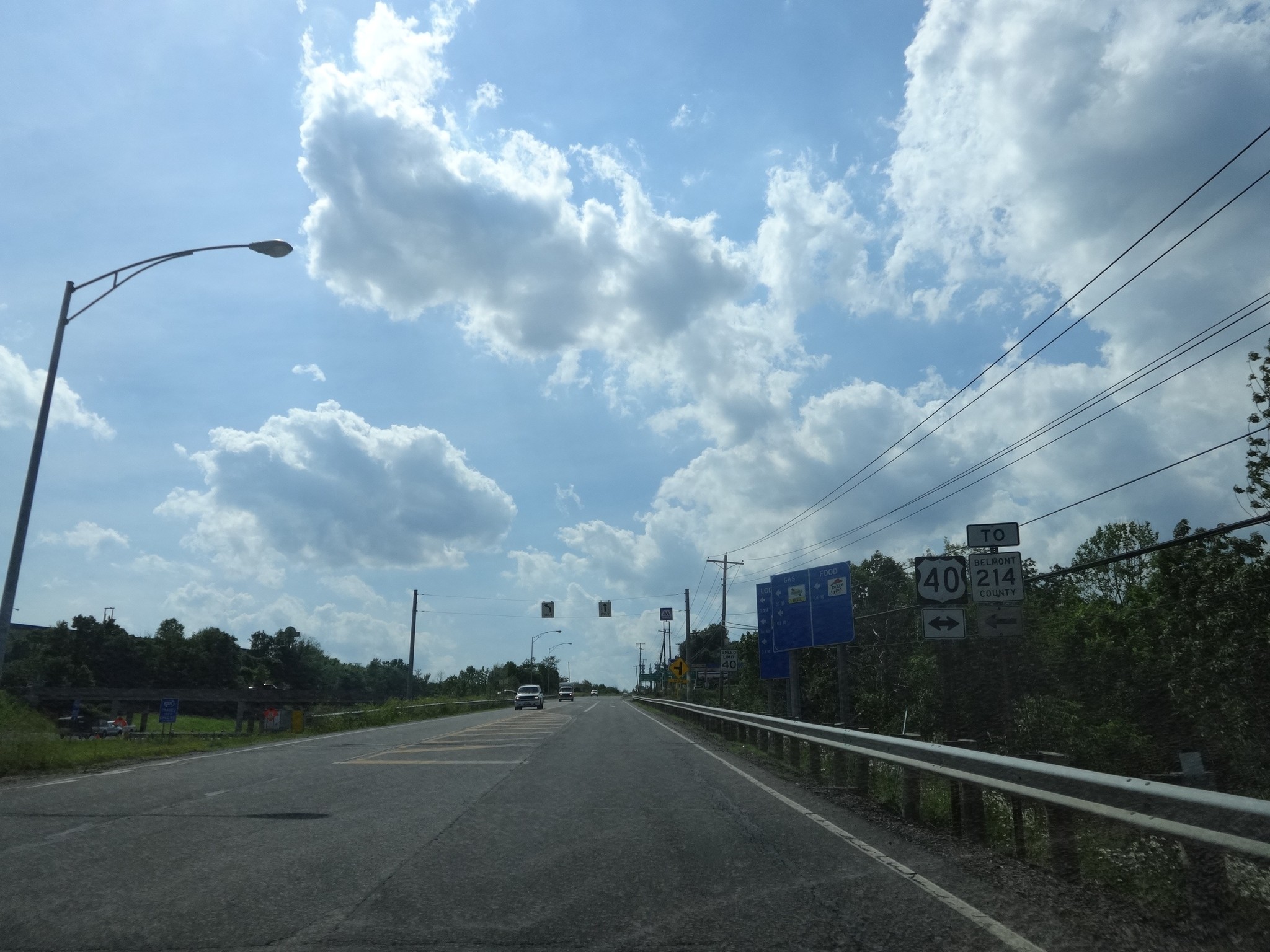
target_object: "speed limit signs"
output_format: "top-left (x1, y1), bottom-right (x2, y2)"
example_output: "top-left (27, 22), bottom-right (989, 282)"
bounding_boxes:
top-left (720, 649), bottom-right (737, 671)
top-left (914, 556), bottom-right (968, 605)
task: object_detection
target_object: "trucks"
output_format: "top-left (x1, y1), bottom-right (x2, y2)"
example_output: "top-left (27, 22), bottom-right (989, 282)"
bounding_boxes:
top-left (558, 683), bottom-right (574, 702)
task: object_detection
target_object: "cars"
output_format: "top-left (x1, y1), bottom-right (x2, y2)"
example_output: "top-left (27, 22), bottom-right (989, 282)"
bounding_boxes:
top-left (622, 693), bottom-right (628, 697)
top-left (514, 685), bottom-right (544, 710)
top-left (497, 690), bottom-right (517, 695)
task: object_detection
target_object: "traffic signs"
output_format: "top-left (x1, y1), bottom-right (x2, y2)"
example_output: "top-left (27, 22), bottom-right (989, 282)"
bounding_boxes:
top-left (770, 560), bottom-right (854, 653)
top-left (541, 603), bottom-right (554, 618)
top-left (598, 602), bottom-right (612, 617)
top-left (756, 583), bottom-right (791, 679)
top-left (967, 522), bottom-right (1025, 639)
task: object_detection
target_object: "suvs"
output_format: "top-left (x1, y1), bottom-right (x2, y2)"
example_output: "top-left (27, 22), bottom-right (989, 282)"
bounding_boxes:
top-left (591, 690), bottom-right (598, 696)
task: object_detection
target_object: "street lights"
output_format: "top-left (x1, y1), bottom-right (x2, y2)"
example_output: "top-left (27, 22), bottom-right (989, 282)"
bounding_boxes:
top-left (530, 630), bottom-right (562, 684)
top-left (547, 643), bottom-right (572, 695)
top-left (1, 238), bottom-right (293, 660)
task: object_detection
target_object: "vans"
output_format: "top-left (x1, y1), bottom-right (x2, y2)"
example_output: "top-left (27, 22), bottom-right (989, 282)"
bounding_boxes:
top-left (57, 716), bottom-right (92, 739)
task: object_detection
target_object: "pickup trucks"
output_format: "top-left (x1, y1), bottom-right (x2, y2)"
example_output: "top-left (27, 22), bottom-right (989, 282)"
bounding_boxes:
top-left (248, 684), bottom-right (283, 691)
top-left (106, 721), bottom-right (136, 734)
top-left (91, 721), bottom-right (123, 738)
